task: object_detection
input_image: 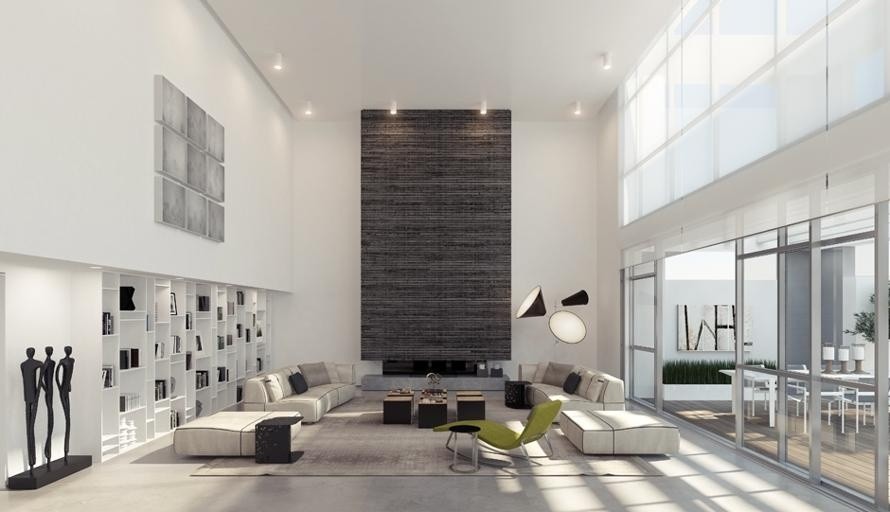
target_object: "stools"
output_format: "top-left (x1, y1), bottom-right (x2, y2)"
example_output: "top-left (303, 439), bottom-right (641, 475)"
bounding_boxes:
top-left (449, 425), bottom-right (480, 472)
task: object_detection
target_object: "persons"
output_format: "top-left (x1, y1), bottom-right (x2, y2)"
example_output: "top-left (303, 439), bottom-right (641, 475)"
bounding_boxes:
top-left (18, 347), bottom-right (44, 467)
top-left (54, 344), bottom-right (76, 456)
top-left (40, 345), bottom-right (56, 462)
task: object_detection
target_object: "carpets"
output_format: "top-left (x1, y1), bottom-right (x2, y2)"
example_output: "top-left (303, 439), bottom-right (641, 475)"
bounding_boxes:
top-left (189, 394), bottom-right (661, 475)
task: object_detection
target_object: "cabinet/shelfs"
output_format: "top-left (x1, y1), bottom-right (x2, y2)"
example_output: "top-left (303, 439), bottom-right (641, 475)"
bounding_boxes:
top-left (361, 375), bottom-right (509, 390)
top-left (101, 269), bottom-right (270, 460)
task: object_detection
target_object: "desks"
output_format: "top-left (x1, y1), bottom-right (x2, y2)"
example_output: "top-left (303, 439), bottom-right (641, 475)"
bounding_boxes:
top-left (419, 399), bottom-right (447, 428)
top-left (456, 397), bottom-right (485, 420)
top-left (383, 396), bottom-right (413, 424)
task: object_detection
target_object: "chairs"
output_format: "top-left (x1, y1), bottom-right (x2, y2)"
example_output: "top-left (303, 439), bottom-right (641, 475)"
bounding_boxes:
top-left (786, 378), bottom-right (845, 434)
top-left (786, 364), bottom-right (808, 416)
top-left (747, 365), bottom-right (778, 417)
top-left (838, 377), bottom-right (877, 435)
top-left (434, 401), bottom-right (562, 466)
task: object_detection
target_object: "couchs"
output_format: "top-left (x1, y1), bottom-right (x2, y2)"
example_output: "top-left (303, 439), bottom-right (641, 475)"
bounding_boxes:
top-left (244, 362), bottom-right (355, 424)
top-left (505, 362), bottom-right (624, 424)
top-left (174, 412), bottom-right (304, 463)
top-left (560, 410), bottom-right (679, 454)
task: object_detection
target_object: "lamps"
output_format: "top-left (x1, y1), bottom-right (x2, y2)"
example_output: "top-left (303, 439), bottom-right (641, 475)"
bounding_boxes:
top-left (549, 311), bottom-right (587, 344)
top-left (561, 290), bottom-right (587, 306)
top-left (516, 286), bottom-right (546, 319)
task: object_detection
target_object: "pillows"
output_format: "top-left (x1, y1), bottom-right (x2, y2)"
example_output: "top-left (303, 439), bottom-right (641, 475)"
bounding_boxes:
top-left (564, 372), bottom-right (581, 394)
top-left (289, 371), bottom-right (307, 394)
